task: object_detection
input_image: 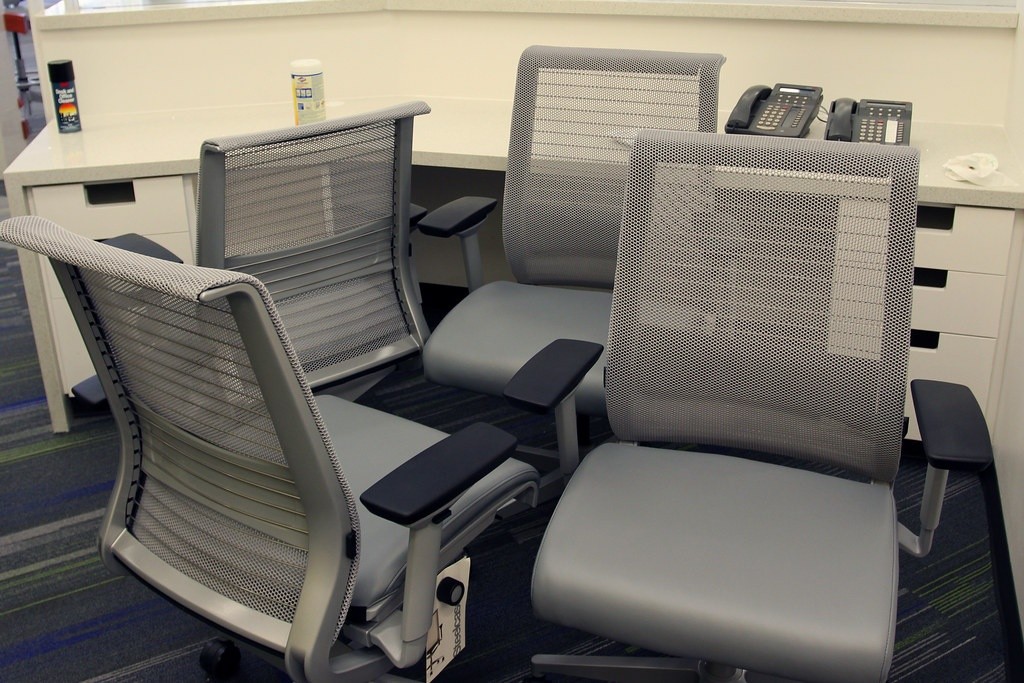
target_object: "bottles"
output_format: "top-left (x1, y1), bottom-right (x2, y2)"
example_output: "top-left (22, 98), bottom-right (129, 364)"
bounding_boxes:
top-left (48, 60), bottom-right (81, 134)
top-left (289, 58), bottom-right (327, 125)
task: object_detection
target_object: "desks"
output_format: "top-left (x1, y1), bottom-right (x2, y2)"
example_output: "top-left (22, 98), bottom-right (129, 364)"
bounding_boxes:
top-left (4, 108), bottom-right (1024, 434)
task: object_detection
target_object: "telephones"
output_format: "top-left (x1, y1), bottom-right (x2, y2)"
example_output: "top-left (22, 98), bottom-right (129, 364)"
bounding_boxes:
top-left (825, 98), bottom-right (913, 145)
top-left (725, 82), bottom-right (823, 137)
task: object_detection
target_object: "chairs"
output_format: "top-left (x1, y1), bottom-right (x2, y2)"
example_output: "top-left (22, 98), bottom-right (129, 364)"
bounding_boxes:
top-left (0, 45), bottom-right (1001, 683)
